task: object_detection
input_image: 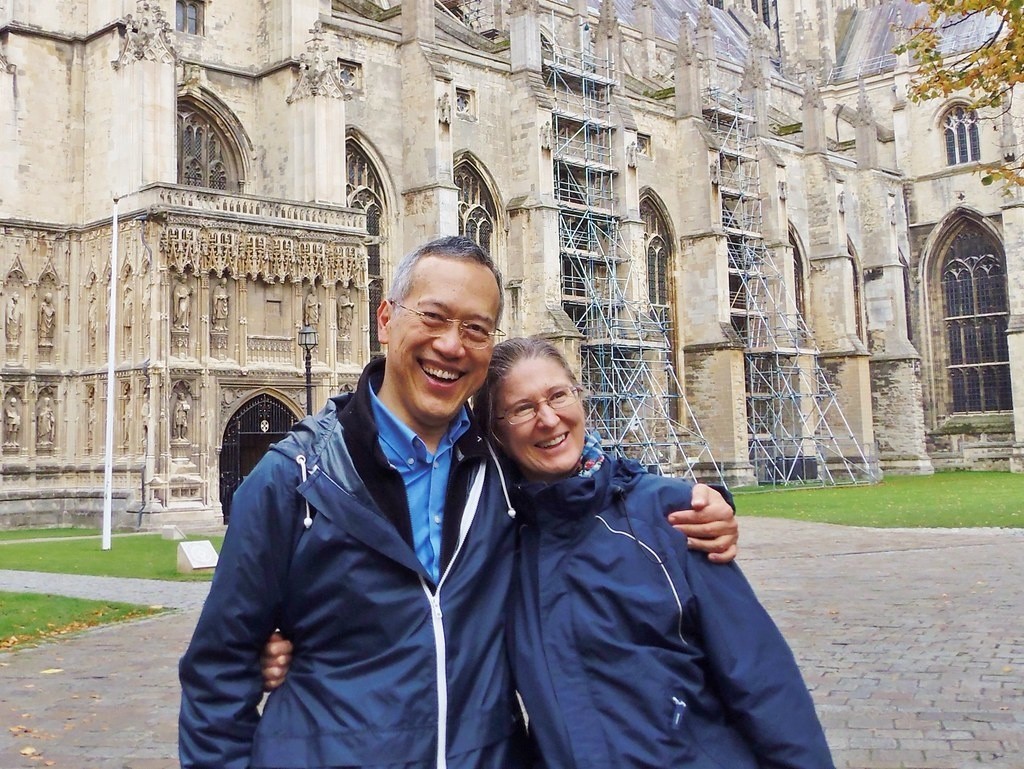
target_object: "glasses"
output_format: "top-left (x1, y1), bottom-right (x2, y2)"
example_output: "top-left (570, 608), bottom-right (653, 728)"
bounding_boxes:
top-left (492, 385), bottom-right (585, 425)
top-left (391, 302), bottom-right (507, 349)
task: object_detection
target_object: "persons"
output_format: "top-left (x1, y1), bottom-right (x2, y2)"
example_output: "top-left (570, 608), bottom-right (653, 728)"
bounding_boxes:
top-left (88, 275), bottom-right (231, 347)
top-left (38, 292), bottom-right (56, 341)
top-left (178, 236), bottom-right (740, 769)
top-left (5, 397), bottom-right (20, 447)
top-left (305, 287), bottom-right (322, 326)
top-left (7, 293), bottom-right (23, 347)
top-left (87, 389), bottom-right (191, 446)
top-left (338, 289), bottom-right (355, 337)
top-left (37, 397), bottom-right (55, 444)
top-left (259, 330), bottom-right (836, 769)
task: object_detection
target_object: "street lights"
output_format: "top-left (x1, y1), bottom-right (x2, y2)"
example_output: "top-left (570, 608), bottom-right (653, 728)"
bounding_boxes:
top-left (297, 324), bottom-right (320, 415)
